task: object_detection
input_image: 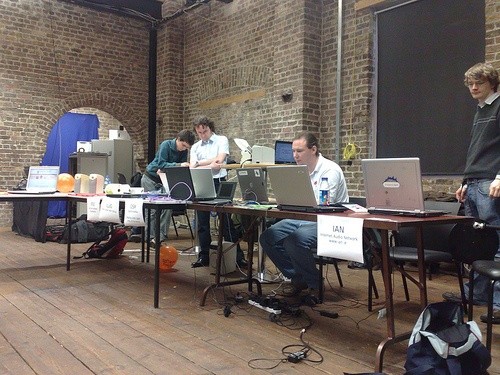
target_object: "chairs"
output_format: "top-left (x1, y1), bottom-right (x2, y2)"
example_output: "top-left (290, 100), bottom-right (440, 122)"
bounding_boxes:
top-left (314, 198), bottom-right (381, 300)
top-left (383, 200), bottom-right (468, 317)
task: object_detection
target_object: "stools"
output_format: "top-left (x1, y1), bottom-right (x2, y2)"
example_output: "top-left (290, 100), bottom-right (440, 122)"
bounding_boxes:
top-left (468, 260), bottom-right (500, 353)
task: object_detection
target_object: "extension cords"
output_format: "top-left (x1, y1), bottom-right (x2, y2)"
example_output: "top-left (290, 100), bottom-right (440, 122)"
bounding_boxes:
top-left (249, 300), bottom-right (281, 315)
top-left (320, 310), bottom-right (338, 318)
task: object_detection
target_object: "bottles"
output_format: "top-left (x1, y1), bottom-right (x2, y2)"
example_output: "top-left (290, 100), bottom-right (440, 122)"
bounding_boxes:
top-left (103, 175), bottom-right (111, 192)
top-left (318, 177), bottom-right (330, 204)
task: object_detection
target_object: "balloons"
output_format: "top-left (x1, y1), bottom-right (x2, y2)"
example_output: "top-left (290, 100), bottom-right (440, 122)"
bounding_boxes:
top-left (57, 173), bottom-right (75, 193)
top-left (158, 245), bottom-right (178, 270)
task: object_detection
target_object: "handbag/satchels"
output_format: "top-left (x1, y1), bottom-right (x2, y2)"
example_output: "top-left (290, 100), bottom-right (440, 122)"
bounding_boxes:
top-left (58, 216), bottom-right (109, 244)
top-left (404, 302), bottom-right (486, 375)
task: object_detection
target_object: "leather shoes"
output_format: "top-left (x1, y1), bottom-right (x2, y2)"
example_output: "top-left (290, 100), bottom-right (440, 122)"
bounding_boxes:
top-left (236, 259), bottom-right (248, 268)
top-left (192, 256), bottom-right (209, 267)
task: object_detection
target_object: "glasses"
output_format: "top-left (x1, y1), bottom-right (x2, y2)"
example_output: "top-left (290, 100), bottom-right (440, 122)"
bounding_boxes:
top-left (465, 79), bottom-right (489, 87)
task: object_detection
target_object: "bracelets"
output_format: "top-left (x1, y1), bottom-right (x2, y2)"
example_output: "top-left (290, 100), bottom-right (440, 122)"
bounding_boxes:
top-left (196, 160), bottom-right (200, 166)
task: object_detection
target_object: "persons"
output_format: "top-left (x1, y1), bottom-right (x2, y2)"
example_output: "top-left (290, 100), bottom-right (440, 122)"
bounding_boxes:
top-left (442, 63), bottom-right (500, 324)
top-left (142, 128), bottom-right (195, 250)
top-left (189, 116), bottom-right (250, 268)
top-left (259, 132), bottom-right (349, 296)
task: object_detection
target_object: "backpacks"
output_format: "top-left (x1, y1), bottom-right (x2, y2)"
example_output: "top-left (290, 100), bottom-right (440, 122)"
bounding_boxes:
top-left (82, 228), bottom-right (128, 259)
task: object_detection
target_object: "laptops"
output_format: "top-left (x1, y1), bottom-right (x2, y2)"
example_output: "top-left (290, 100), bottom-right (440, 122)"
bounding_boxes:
top-left (163, 158), bottom-right (447, 217)
top-left (8, 166), bottom-right (61, 195)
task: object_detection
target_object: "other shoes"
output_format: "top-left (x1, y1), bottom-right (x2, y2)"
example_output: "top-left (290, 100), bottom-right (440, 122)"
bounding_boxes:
top-left (301, 290), bottom-right (322, 305)
top-left (480, 310), bottom-right (500, 323)
top-left (150, 239), bottom-right (157, 248)
top-left (443, 292), bottom-right (478, 304)
top-left (284, 280), bottom-right (308, 294)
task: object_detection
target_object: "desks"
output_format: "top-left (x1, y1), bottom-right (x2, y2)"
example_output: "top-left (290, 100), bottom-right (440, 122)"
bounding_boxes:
top-left (0, 178), bottom-right (477, 372)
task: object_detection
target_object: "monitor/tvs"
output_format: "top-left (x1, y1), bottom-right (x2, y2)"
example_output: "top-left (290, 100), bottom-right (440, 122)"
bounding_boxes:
top-left (274, 141), bottom-right (296, 164)
